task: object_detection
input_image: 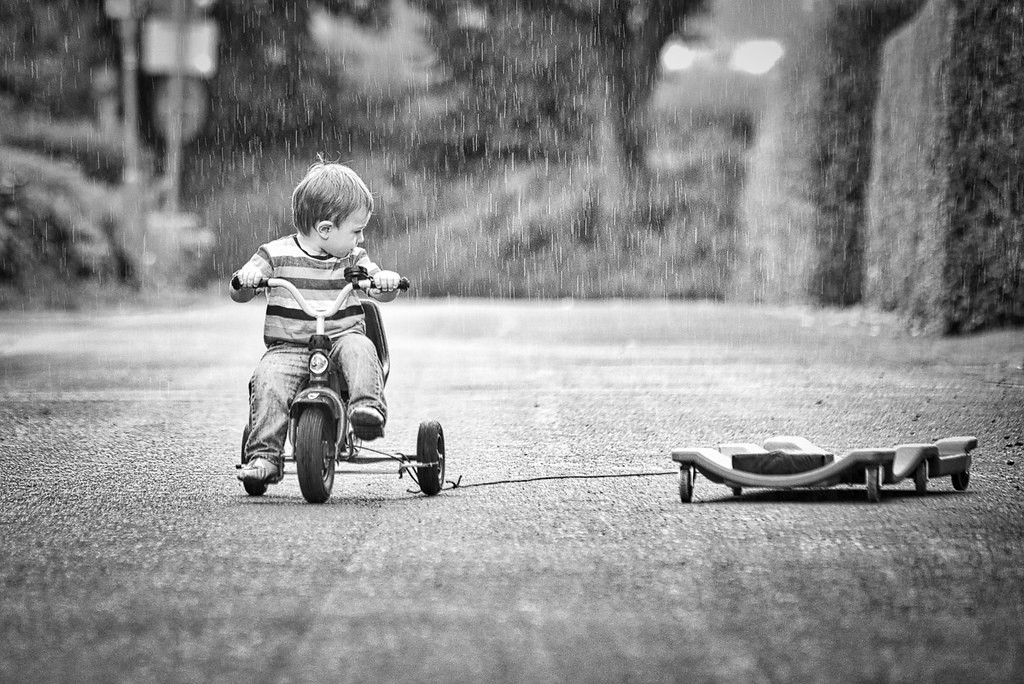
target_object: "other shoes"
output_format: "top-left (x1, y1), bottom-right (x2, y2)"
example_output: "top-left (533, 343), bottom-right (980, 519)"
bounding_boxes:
top-left (350, 407), bottom-right (386, 441)
top-left (239, 456), bottom-right (280, 481)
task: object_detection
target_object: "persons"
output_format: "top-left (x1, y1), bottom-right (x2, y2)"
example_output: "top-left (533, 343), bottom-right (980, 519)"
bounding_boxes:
top-left (229, 162), bottom-right (401, 482)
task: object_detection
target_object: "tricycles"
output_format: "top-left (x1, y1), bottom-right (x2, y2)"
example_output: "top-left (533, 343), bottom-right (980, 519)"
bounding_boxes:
top-left (230, 264), bottom-right (446, 506)
